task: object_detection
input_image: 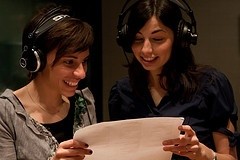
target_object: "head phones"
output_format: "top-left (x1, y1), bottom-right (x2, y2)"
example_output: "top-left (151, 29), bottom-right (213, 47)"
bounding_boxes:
top-left (18, 5), bottom-right (73, 76)
top-left (116, 0), bottom-right (198, 54)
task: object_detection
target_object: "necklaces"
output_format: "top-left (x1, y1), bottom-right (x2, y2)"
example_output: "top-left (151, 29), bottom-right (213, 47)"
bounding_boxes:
top-left (26, 87), bottom-right (63, 115)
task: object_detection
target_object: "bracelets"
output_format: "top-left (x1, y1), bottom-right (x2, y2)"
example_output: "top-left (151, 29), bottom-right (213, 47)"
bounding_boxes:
top-left (213, 152), bottom-right (217, 160)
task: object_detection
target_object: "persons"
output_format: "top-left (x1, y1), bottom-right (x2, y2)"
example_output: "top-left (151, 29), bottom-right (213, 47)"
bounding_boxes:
top-left (0, 11), bottom-right (98, 160)
top-left (106, 0), bottom-right (240, 160)
